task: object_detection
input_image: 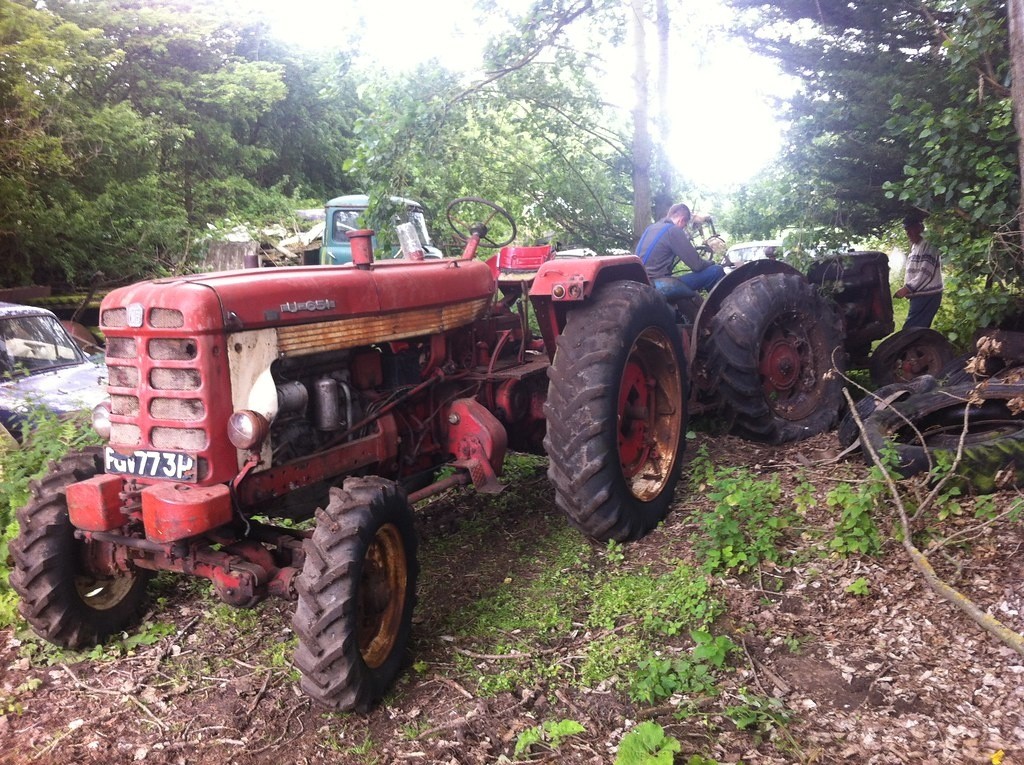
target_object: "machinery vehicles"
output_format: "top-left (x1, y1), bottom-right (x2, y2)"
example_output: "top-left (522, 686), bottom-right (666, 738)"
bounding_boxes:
top-left (8, 197), bottom-right (905, 717)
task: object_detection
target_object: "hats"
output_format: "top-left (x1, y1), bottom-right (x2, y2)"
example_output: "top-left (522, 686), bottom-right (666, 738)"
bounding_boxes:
top-left (902, 214), bottom-right (922, 228)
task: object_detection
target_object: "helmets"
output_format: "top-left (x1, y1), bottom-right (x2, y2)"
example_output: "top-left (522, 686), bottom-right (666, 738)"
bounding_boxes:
top-left (766, 246), bottom-right (783, 257)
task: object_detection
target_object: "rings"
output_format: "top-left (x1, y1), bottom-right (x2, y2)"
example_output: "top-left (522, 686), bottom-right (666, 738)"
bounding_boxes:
top-left (901, 296), bottom-right (902, 297)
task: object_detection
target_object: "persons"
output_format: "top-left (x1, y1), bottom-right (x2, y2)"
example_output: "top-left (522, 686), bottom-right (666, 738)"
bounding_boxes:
top-left (636, 203), bottom-right (726, 292)
top-left (893, 214), bottom-right (943, 372)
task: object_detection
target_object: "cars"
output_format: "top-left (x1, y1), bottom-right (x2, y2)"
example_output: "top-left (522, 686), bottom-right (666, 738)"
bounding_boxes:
top-left (0, 301), bottom-right (108, 444)
top-left (720, 239), bottom-right (811, 275)
